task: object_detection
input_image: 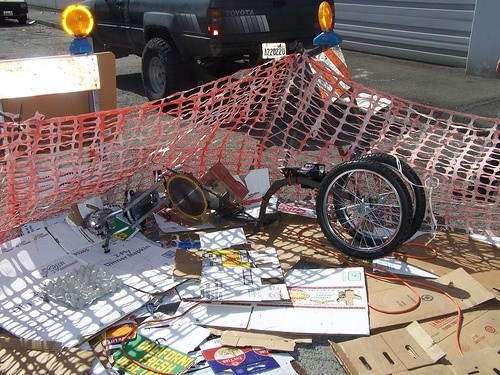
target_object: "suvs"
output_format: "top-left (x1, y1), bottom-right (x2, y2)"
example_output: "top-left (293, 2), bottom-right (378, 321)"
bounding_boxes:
top-left (87, 1), bottom-right (340, 110)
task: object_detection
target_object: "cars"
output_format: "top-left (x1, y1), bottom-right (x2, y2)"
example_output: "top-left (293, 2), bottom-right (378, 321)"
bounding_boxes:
top-left (0, 0), bottom-right (29, 25)
